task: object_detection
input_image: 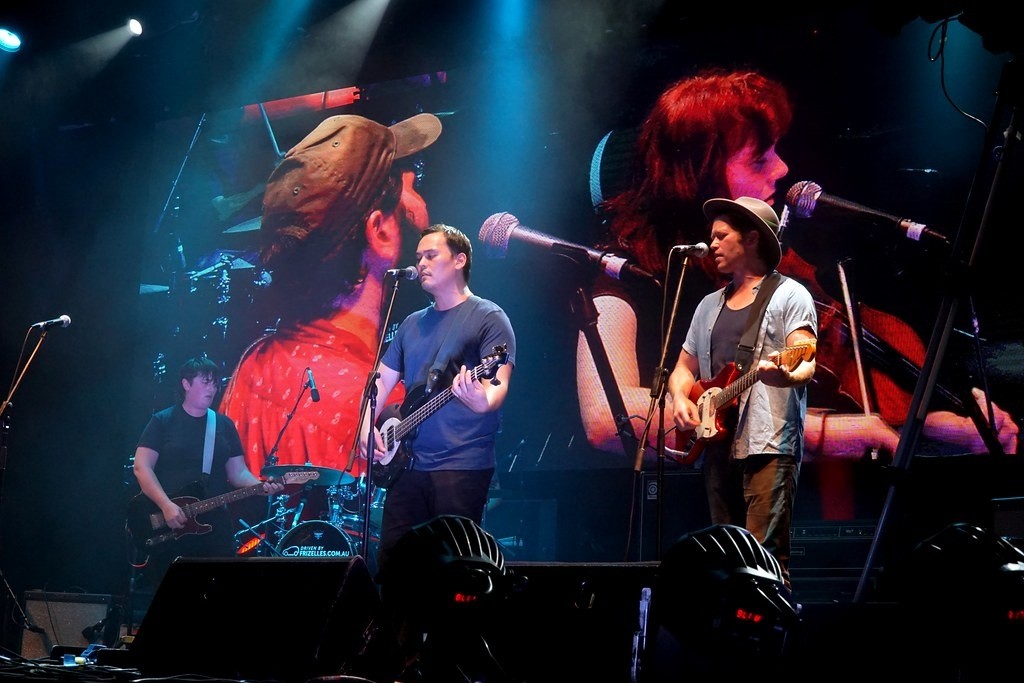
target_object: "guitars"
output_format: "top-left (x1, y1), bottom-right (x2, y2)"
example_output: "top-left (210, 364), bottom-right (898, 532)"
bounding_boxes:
top-left (675, 338), bottom-right (818, 466)
top-left (371, 343), bottom-right (510, 490)
top-left (127, 469), bottom-right (320, 559)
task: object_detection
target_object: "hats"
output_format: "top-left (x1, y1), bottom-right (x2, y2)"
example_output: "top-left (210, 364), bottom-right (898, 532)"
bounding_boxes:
top-left (702, 196), bottom-right (781, 272)
top-left (259, 114), bottom-right (443, 284)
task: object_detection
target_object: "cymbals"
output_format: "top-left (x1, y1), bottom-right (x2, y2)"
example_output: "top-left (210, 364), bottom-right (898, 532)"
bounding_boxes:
top-left (220, 215), bottom-right (262, 234)
top-left (260, 464), bottom-right (355, 486)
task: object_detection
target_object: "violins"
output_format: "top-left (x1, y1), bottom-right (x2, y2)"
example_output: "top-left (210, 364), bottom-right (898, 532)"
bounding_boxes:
top-left (774, 239), bottom-right (968, 424)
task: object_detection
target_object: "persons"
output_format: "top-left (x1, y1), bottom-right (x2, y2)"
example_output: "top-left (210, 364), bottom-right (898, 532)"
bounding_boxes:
top-left (576, 72), bottom-right (1019, 530)
top-left (215, 111), bottom-right (444, 557)
top-left (668, 197), bottom-right (818, 558)
top-left (132, 356), bottom-right (286, 629)
top-left (358, 225), bottom-right (516, 587)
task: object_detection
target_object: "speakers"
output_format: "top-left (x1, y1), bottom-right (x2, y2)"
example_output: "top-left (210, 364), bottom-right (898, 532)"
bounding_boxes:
top-left (640, 471), bottom-right (713, 563)
top-left (488, 561), bottom-right (662, 683)
top-left (783, 599), bottom-right (896, 683)
top-left (19, 590), bottom-right (120, 662)
top-left (127, 553), bottom-right (378, 681)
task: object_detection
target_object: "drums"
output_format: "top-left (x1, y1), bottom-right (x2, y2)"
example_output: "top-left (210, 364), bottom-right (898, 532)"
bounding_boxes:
top-left (271, 520), bottom-right (381, 575)
top-left (321, 511), bottom-right (381, 544)
top-left (363, 483), bottom-right (388, 528)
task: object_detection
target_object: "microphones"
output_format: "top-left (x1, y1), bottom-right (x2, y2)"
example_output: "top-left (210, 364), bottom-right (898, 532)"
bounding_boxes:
top-left (388, 266), bottom-right (418, 279)
top-left (177, 238), bottom-right (186, 268)
top-left (308, 368), bottom-right (320, 402)
top-left (82, 619), bottom-right (105, 637)
top-left (478, 211), bottom-right (655, 283)
top-left (784, 181), bottom-right (947, 244)
top-left (23, 626), bottom-right (45, 633)
top-left (673, 243), bottom-right (709, 258)
top-left (31, 315), bottom-right (71, 329)
top-left (291, 497), bottom-right (307, 528)
